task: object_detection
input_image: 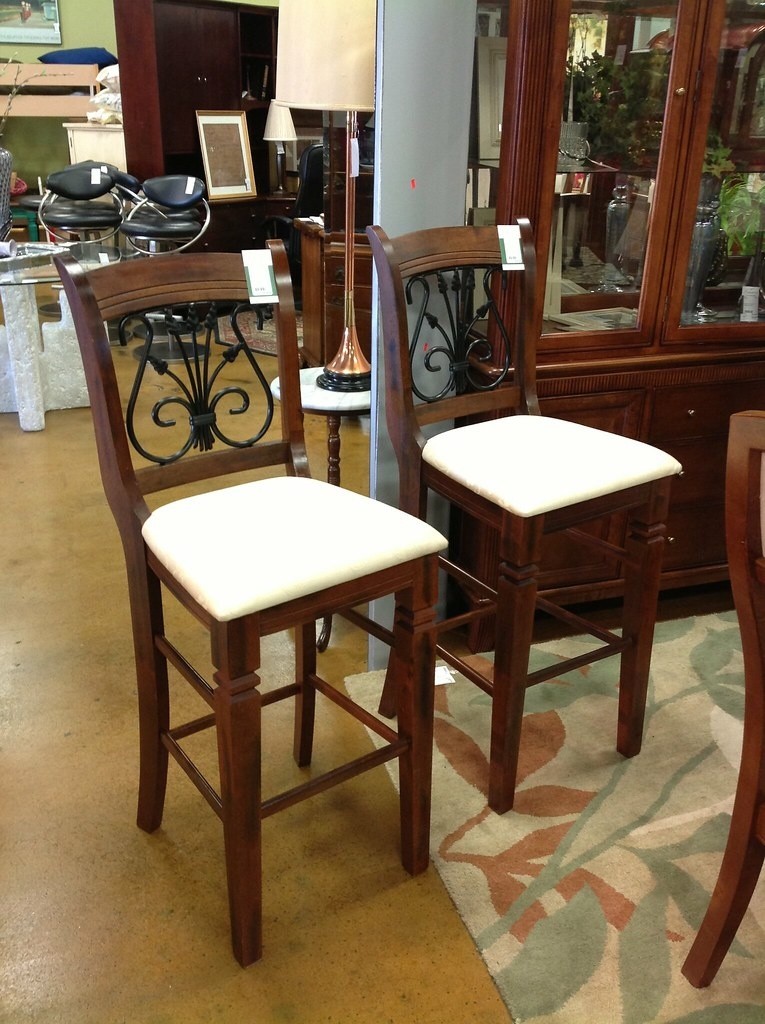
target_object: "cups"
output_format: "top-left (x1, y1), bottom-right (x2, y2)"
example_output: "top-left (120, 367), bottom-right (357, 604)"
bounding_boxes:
top-left (558, 121), bottom-right (589, 166)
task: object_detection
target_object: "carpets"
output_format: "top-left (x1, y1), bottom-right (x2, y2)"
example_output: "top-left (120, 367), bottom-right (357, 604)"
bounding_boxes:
top-left (214, 310), bottom-right (303, 359)
top-left (351, 606), bottom-right (765, 1024)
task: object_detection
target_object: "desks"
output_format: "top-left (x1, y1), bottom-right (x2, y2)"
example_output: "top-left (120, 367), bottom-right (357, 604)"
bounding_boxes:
top-left (269, 368), bottom-right (371, 487)
top-left (0, 242), bottom-right (122, 435)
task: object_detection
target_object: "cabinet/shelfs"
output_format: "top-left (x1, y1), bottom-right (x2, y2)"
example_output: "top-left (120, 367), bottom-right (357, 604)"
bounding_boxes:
top-left (62, 123), bottom-right (127, 249)
top-left (115, 0), bottom-right (279, 317)
top-left (293, 217), bottom-right (373, 367)
top-left (445, 0), bottom-right (765, 648)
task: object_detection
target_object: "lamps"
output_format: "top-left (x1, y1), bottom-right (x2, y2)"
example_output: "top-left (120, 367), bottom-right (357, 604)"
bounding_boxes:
top-left (274, 0), bottom-right (378, 393)
top-left (263, 100), bottom-right (299, 197)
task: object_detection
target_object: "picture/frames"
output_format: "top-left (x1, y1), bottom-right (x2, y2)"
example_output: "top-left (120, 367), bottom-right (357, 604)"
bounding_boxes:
top-left (195, 109), bottom-right (259, 199)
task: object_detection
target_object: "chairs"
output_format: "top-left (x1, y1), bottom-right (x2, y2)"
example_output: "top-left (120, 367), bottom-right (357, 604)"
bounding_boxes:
top-left (363, 214), bottom-right (686, 816)
top-left (678, 409), bottom-right (765, 993)
top-left (51, 236), bottom-right (451, 966)
top-left (256, 143), bottom-right (323, 332)
